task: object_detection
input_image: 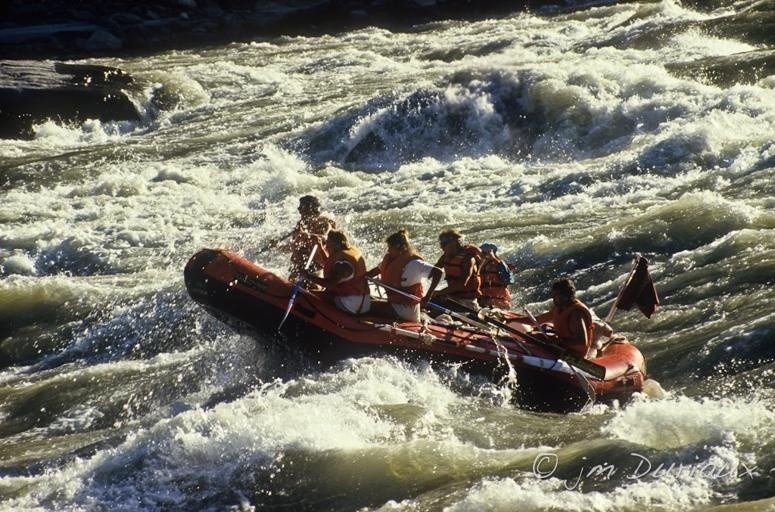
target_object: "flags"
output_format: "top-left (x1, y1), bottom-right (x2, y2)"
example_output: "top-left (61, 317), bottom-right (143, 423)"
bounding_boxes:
top-left (616, 257), bottom-right (660, 319)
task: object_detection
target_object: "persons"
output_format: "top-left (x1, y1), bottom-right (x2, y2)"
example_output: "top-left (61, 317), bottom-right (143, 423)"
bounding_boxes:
top-left (289, 195), bottom-right (515, 321)
top-left (500, 277), bottom-right (593, 359)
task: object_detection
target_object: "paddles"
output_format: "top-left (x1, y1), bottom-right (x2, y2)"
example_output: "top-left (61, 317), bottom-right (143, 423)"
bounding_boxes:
top-left (279, 244), bottom-right (320, 331)
top-left (444, 296), bottom-right (606, 380)
top-left (524, 308), bottom-right (596, 401)
top-left (365, 275), bottom-right (490, 332)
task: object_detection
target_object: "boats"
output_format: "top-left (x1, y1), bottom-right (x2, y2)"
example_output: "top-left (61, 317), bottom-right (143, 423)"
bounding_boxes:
top-left (183, 248), bottom-right (649, 412)
